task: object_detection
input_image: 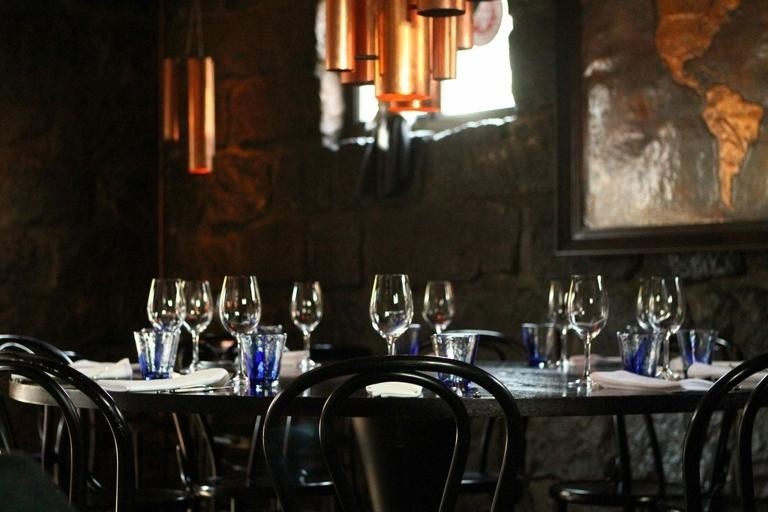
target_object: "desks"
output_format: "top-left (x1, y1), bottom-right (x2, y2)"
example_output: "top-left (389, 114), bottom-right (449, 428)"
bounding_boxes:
top-left (8, 362), bottom-right (767, 510)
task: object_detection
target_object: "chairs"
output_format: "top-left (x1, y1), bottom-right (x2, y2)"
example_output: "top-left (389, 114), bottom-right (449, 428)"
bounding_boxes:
top-left (1, 328), bottom-right (767, 511)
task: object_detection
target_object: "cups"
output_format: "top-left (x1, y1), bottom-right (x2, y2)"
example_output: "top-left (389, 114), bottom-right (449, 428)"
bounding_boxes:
top-left (617, 331), bottom-right (663, 380)
top-left (430, 333), bottom-right (480, 396)
top-left (521, 321), bottom-right (562, 368)
top-left (675, 328), bottom-right (717, 383)
top-left (243, 322), bottom-right (288, 400)
top-left (132, 328), bottom-right (181, 381)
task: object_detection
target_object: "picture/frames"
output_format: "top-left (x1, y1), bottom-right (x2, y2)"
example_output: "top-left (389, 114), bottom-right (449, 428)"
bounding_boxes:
top-left (557, 1), bottom-right (766, 250)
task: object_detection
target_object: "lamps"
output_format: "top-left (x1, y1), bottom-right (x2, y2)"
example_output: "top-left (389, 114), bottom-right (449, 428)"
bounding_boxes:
top-left (324, 1), bottom-right (475, 113)
top-left (157, 2), bottom-right (219, 174)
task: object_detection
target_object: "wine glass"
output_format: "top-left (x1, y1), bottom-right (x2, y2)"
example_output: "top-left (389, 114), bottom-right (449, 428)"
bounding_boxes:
top-left (291, 280), bottom-right (324, 376)
top-left (368, 274), bottom-right (454, 400)
top-left (636, 275), bottom-right (684, 383)
top-left (144, 278), bottom-right (213, 374)
top-left (217, 274), bottom-right (262, 394)
top-left (546, 274), bottom-right (610, 393)
